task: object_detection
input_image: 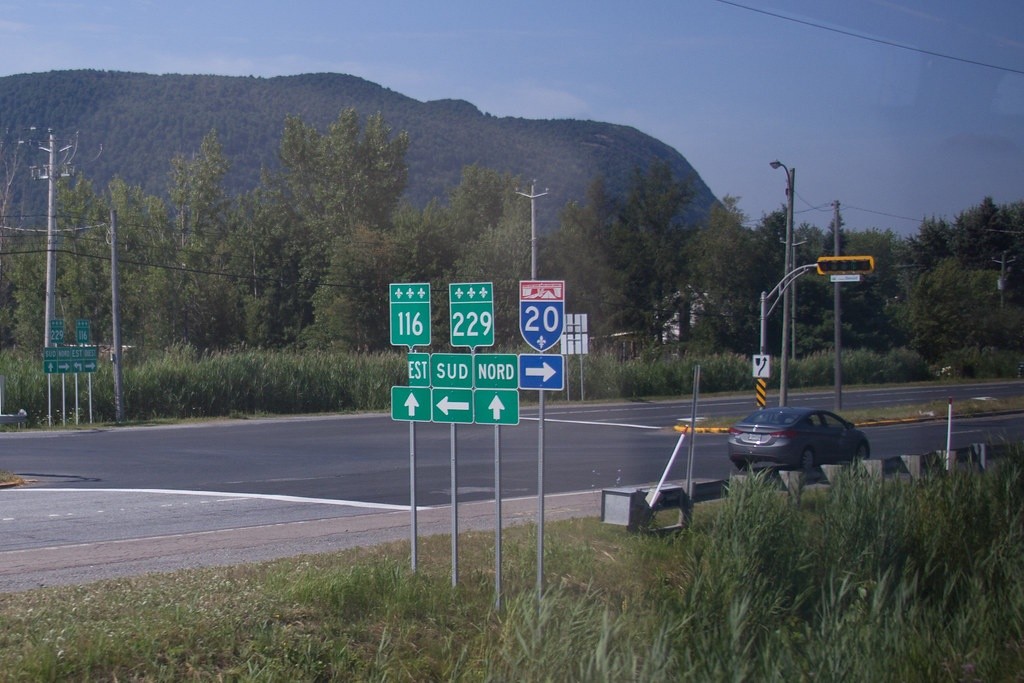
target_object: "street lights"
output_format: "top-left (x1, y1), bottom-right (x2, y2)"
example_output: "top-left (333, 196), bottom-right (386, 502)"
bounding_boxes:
top-left (769, 158), bottom-right (796, 407)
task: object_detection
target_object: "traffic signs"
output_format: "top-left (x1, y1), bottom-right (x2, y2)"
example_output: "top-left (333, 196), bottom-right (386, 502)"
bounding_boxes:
top-left (389, 280), bottom-right (566, 425)
top-left (44, 319), bottom-right (99, 373)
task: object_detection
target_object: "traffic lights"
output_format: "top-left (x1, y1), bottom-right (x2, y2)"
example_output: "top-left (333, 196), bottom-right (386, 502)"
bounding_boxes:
top-left (817, 256), bottom-right (874, 275)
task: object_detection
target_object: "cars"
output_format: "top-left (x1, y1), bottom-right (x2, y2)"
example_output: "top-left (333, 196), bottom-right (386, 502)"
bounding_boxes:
top-left (727, 407), bottom-right (872, 471)
